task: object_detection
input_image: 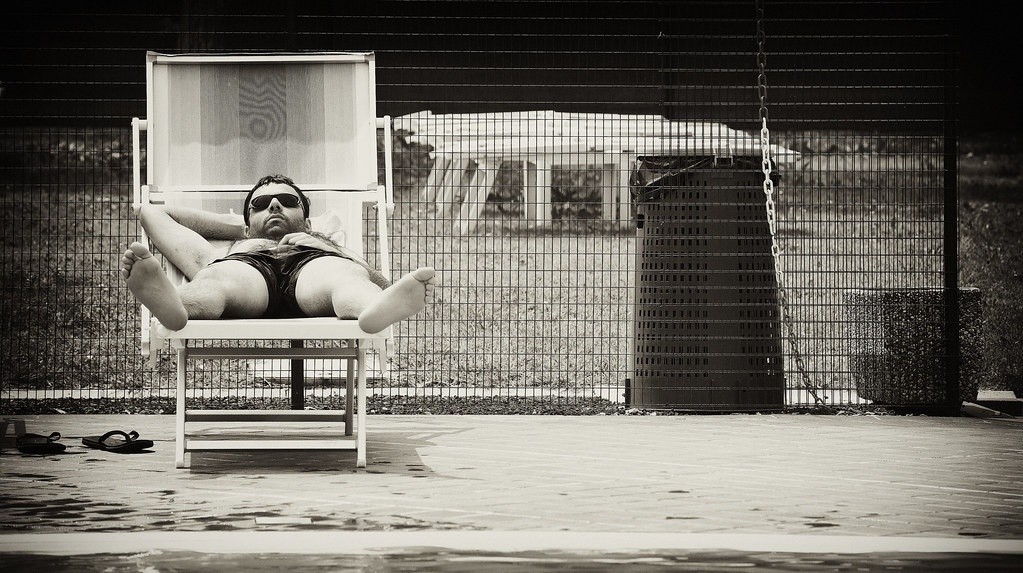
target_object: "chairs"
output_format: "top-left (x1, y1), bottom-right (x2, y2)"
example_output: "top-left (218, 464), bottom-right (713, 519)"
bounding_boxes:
top-left (130, 49), bottom-right (394, 471)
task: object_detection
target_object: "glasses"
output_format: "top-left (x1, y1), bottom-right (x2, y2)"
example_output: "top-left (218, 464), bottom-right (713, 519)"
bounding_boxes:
top-left (246, 193), bottom-right (305, 223)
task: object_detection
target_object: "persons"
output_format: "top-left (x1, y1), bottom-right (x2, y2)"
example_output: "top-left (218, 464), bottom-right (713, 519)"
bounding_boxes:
top-left (121, 175), bottom-right (438, 334)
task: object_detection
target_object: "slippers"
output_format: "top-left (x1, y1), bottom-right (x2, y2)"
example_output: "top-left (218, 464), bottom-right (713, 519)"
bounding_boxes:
top-left (82, 430), bottom-right (154, 451)
top-left (15, 432), bottom-right (66, 454)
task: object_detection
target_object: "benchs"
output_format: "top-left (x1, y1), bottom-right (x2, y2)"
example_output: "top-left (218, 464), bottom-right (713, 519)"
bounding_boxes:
top-left (393, 110), bottom-right (802, 236)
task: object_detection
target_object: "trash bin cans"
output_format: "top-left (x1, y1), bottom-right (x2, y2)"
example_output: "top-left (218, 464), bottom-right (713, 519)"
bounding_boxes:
top-left (626, 151), bottom-right (786, 416)
